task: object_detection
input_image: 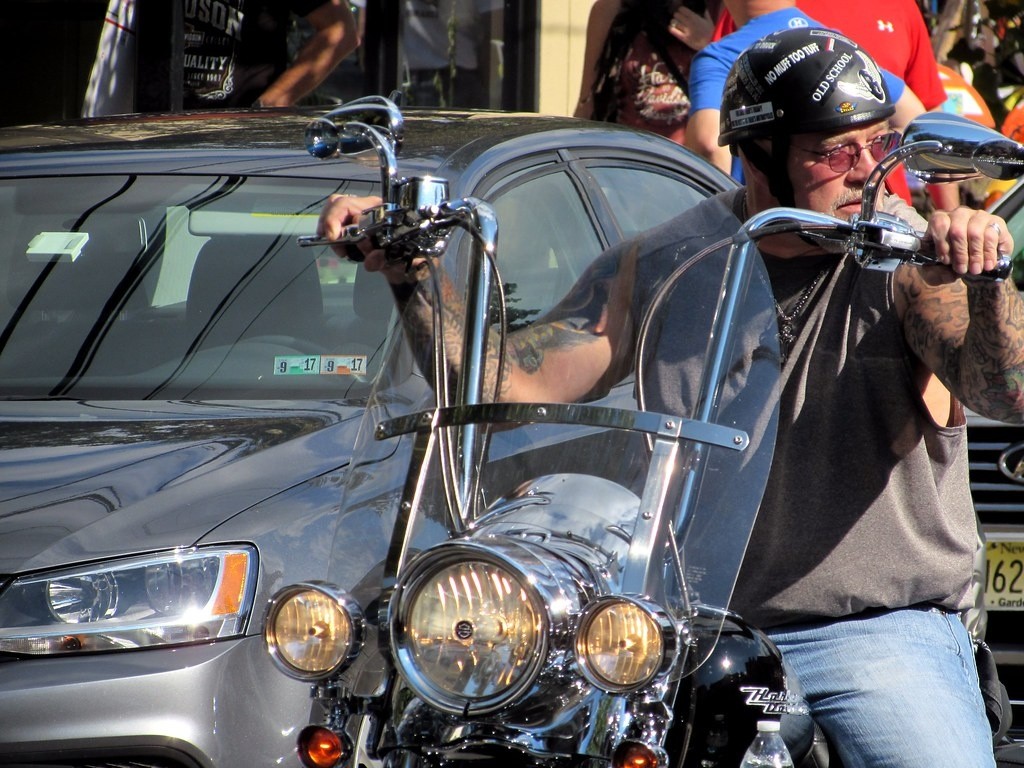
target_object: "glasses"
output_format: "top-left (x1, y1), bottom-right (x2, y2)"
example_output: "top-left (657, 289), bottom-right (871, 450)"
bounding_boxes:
top-left (798, 128), bottom-right (902, 173)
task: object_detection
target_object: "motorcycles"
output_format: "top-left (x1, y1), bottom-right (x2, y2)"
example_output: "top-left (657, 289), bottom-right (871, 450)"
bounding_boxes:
top-left (261, 98), bottom-right (1024, 768)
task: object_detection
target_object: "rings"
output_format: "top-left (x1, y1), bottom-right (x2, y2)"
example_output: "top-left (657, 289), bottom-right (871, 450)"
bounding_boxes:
top-left (989, 222), bottom-right (1001, 235)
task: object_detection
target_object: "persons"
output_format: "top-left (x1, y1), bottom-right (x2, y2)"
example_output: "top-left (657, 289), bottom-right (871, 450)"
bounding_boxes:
top-left (314, 29), bottom-right (1024, 768)
top-left (183, 0), bottom-right (483, 108)
top-left (587, 0), bottom-right (1023, 205)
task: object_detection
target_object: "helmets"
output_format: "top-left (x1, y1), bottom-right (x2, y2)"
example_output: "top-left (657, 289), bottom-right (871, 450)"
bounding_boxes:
top-left (717, 27), bottom-right (897, 147)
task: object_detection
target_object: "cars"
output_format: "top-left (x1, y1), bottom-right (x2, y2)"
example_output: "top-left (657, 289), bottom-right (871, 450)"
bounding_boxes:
top-left (1, 110), bottom-right (752, 767)
top-left (947, 163), bottom-right (1024, 759)
